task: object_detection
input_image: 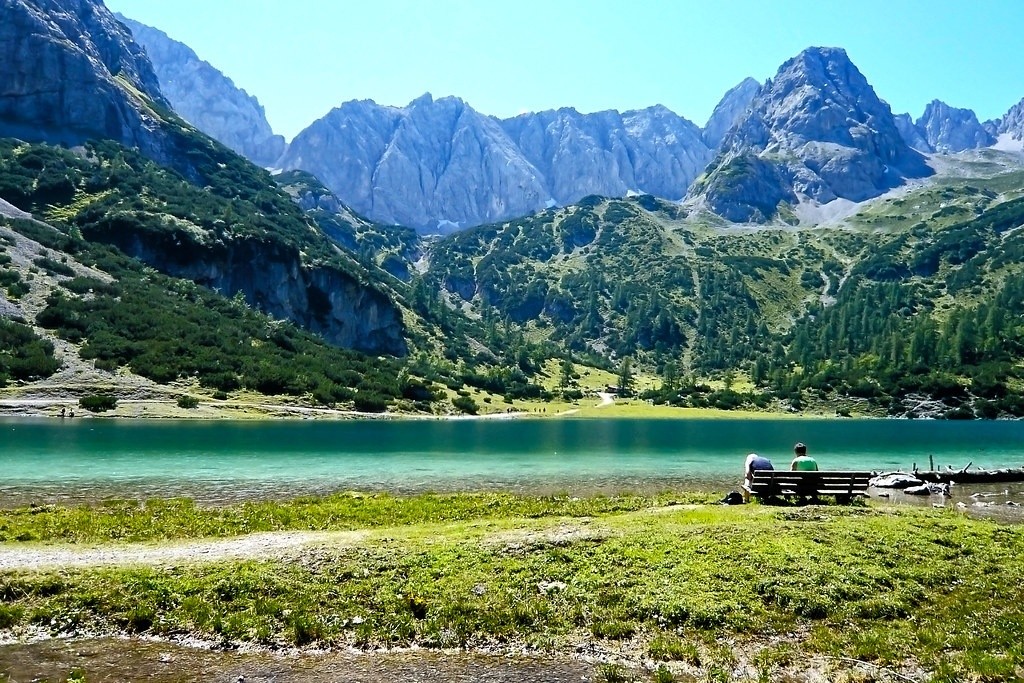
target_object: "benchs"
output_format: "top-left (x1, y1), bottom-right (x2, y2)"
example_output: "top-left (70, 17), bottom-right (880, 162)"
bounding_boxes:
top-left (741, 470), bottom-right (870, 505)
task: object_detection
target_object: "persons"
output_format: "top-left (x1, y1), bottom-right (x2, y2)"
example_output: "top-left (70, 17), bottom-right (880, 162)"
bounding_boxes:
top-left (792, 443), bottom-right (818, 492)
top-left (745, 454), bottom-right (774, 485)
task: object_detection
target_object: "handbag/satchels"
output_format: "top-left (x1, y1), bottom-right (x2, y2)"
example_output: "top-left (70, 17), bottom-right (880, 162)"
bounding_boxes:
top-left (721, 489), bottom-right (743, 505)
